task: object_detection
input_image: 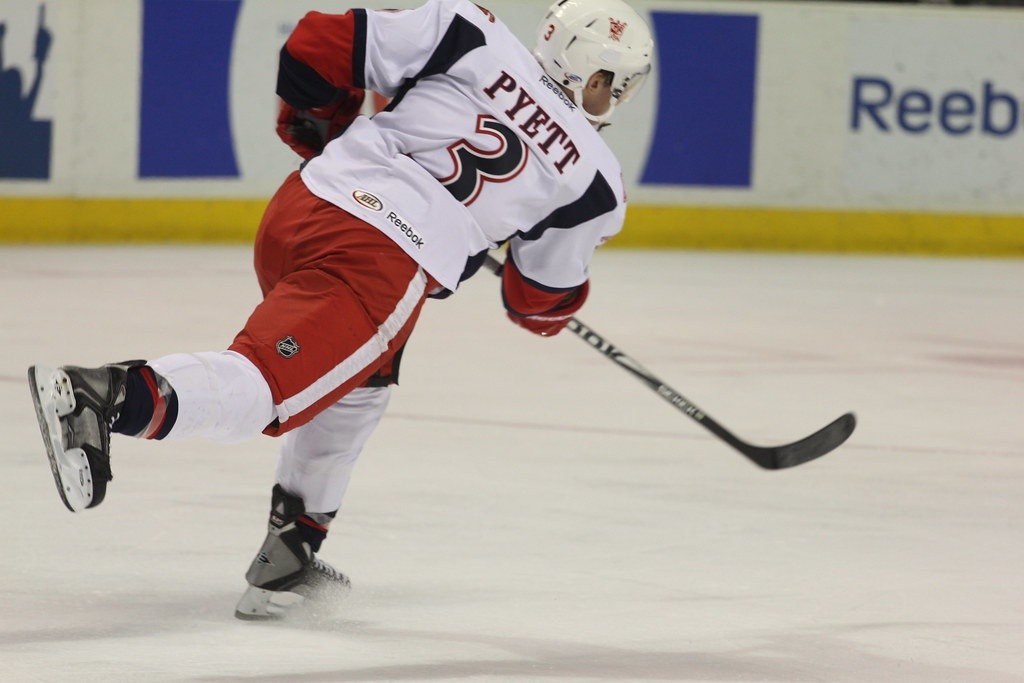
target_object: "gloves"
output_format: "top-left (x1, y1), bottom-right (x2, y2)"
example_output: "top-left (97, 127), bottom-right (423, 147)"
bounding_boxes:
top-left (276, 85), bottom-right (366, 163)
top-left (508, 276), bottom-right (590, 338)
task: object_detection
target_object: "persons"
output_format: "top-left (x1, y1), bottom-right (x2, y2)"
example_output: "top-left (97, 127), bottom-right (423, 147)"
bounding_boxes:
top-left (25, 0), bottom-right (654, 622)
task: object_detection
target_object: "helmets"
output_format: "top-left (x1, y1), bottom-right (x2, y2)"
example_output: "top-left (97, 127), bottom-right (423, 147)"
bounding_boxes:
top-left (534, 0), bottom-right (654, 121)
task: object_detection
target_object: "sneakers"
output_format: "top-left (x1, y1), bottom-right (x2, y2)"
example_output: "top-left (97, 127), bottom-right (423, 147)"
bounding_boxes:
top-left (235, 482), bottom-right (353, 621)
top-left (28, 360), bottom-right (147, 511)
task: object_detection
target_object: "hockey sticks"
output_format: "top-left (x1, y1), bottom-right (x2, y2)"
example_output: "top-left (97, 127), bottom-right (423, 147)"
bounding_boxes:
top-left (481, 252), bottom-right (858, 472)
